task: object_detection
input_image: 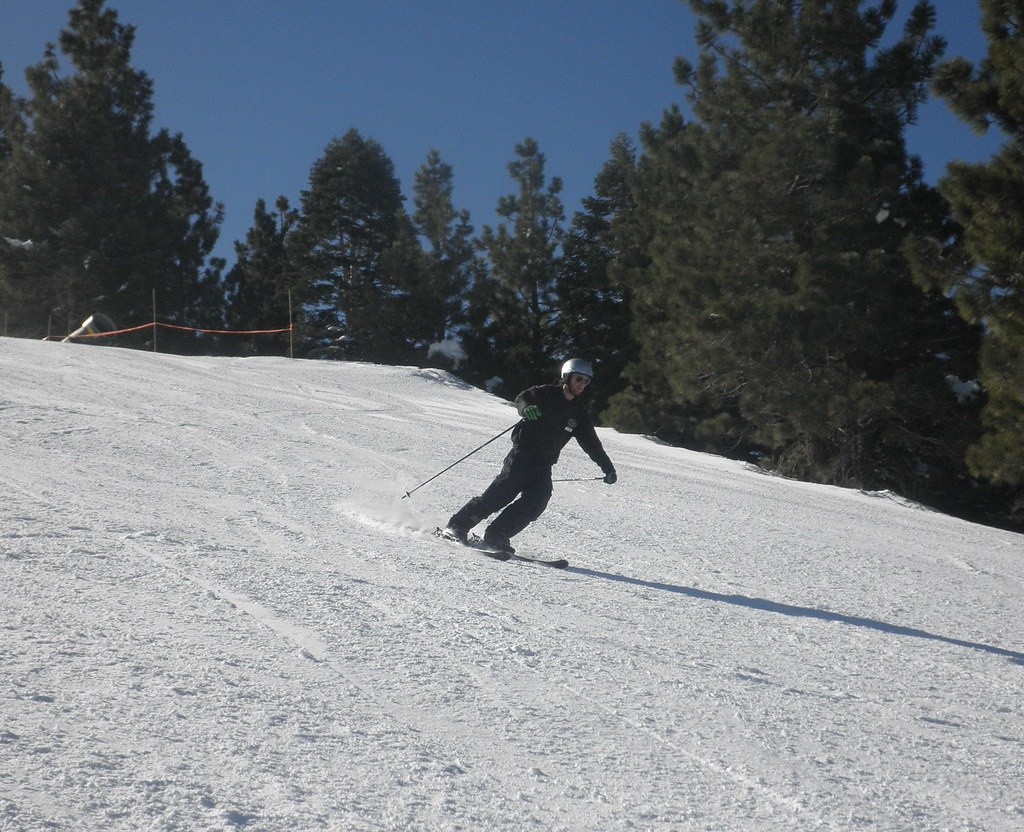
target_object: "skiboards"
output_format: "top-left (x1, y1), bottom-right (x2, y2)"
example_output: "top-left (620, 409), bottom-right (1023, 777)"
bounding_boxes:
top-left (433, 525), bottom-right (570, 569)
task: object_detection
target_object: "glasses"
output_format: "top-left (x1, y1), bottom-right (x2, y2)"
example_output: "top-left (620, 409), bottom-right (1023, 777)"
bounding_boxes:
top-left (571, 373), bottom-right (591, 385)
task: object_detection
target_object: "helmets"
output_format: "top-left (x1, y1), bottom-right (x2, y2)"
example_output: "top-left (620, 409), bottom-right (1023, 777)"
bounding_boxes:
top-left (561, 358), bottom-right (594, 379)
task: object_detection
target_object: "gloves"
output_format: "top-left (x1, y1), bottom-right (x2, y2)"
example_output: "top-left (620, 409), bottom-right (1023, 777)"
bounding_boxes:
top-left (523, 405), bottom-right (542, 421)
top-left (603, 473), bottom-right (617, 485)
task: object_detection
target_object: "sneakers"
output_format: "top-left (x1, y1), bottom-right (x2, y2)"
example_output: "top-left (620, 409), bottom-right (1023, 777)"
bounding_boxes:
top-left (442, 525), bottom-right (469, 546)
top-left (478, 533), bottom-right (516, 554)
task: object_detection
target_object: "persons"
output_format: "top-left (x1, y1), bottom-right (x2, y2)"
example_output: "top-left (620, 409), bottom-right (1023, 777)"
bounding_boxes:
top-left (442, 358), bottom-right (618, 554)
